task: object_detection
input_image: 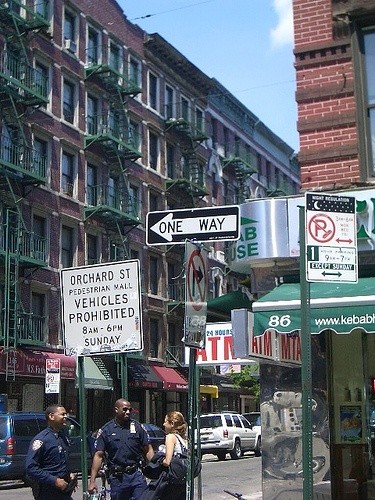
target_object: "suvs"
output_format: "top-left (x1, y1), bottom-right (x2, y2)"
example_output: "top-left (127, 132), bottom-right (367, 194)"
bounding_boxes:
top-left (194, 410), bottom-right (262, 460)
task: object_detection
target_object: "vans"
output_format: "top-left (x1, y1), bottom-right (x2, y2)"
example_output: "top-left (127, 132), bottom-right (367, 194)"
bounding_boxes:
top-left (0, 410), bottom-right (94, 485)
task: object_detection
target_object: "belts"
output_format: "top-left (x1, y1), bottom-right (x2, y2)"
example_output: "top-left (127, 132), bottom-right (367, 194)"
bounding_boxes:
top-left (113, 463), bottom-right (139, 474)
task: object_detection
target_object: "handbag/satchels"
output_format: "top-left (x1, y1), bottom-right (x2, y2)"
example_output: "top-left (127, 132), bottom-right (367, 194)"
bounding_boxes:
top-left (169, 445), bottom-right (202, 483)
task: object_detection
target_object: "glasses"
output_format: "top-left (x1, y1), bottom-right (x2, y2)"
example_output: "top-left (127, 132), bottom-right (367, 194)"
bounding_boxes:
top-left (118, 407), bottom-right (133, 411)
top-left (52, 412), bottom-right (68, 416)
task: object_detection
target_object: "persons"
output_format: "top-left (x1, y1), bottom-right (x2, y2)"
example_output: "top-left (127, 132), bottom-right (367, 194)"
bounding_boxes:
top-left (26, 405), bottom-right (78, 499)
top-left (137, 410), bottom-right (192, 500)
top-left (89, 398), bottom-right (156, 500)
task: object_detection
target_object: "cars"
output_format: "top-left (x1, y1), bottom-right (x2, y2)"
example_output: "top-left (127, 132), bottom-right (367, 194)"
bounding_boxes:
top-left (141, 423), bottom-right (167, 452)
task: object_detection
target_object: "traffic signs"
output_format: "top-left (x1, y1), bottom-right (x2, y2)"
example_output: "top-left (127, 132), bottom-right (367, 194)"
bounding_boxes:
top-left (304, 191), bottom-right (359, 285)
top-left (60, 259), bottom-right (144, 356)
top-left (146, 205), bottom-right (241, 246)
